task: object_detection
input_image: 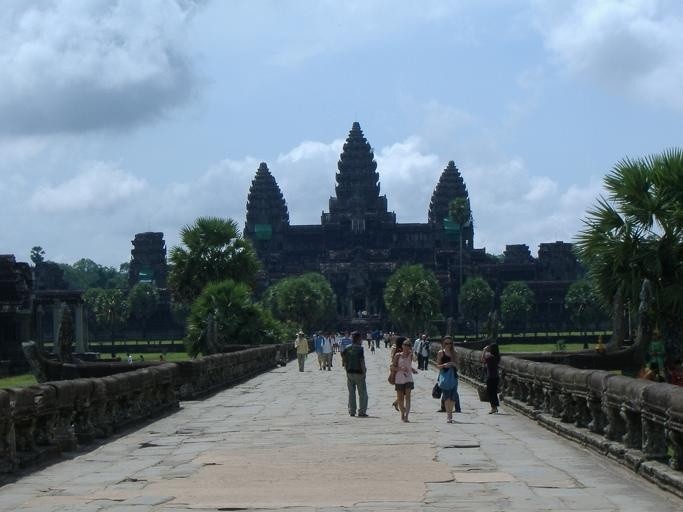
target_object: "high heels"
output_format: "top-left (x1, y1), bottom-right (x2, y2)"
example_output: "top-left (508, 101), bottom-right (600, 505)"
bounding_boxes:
top-left (489, 409), bottom-right (498, 414)
top-left (447, 412), bottom-right (452, 423)
top-left (392, 402), bottom-right (399, 411)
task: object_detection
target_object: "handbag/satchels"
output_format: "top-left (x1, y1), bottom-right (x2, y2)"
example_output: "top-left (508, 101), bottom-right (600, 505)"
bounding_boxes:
top-left (477, 387), bottom-right (491, 402)
top-left (432, 383), bottom-right (442, 399)
top-left (438, 368), bottom-right (456, 390)
top-left (388, 352), bottom-right (400, 384)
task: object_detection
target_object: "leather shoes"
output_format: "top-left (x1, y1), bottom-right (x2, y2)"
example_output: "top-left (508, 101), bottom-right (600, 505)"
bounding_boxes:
top-left (437, 408), bottom-right (446, 412)
top-left (358, 413), bottom-right (369, 417)
top-left (452, 410), bottom-right (461, 413)
top-left (349, 413), bottom-right (355, 416)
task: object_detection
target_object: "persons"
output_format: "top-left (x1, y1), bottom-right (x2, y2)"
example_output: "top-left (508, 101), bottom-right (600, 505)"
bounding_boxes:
top-left (390, 338), bottom-right (418, 423)
top-left (480, 343), bottom-right (501, 414)
top-left (437, 336), bottom-right (461, 423)
top-left (342, 331), bottom-right (368, 416)
top-left (295, 328), bottom-right (431, 411)
top-left (643, 327), bottom-right (668, 381)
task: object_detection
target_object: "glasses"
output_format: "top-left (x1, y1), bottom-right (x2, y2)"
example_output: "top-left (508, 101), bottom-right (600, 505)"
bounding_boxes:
top-left (403, 344), bottom-right (411, 347)
top-left (444, 342), bottom-right (453, 345)
